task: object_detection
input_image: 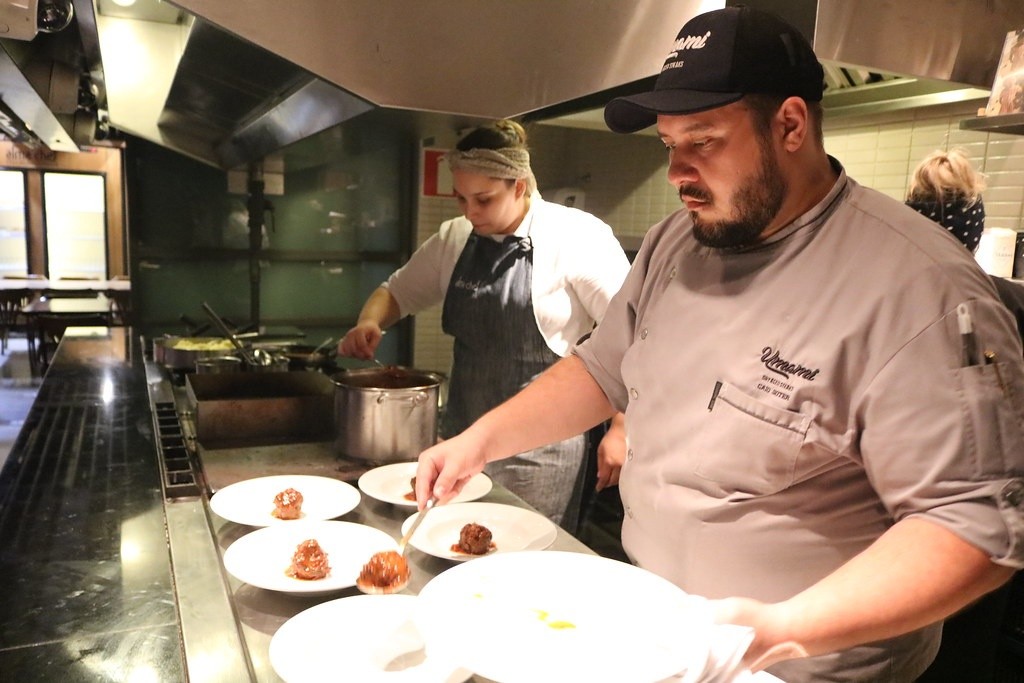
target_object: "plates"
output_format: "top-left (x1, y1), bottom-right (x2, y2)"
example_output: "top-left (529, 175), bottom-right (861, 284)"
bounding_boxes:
top-left (358, 462), bottom-right (493, 507)
top-left (412, 550), bottom-right (692, 683)
top-left (267, 595), bottom-right (473, 683)
top-left (401, 503), bottom-right (557, 561)
top-left (223, 520), bottom-right (399, 594)
top-left (209, 475), bottom-right (361, 527)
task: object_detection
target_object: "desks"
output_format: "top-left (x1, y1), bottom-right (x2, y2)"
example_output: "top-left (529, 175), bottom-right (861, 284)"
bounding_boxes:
top-left (0, 279), bottom-right (131, 354)
top-left (20, 297), bottom-right (132, 387)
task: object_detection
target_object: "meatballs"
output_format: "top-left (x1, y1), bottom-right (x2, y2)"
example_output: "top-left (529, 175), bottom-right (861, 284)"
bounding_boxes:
top-left (459, 523), bottom-right (493, 554)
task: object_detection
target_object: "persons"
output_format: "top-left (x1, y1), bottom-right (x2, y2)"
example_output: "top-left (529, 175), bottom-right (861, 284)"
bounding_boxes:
top-left (902, 146), bottom-right (1024, 346)
top-left (340, 118), bottom-right (630, 524)
top-left (413, 9), bottom-right (1023, 682)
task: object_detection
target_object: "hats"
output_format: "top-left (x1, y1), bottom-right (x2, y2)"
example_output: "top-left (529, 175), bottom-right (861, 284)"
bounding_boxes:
top-left (603, 8), bottom-right (824, 134)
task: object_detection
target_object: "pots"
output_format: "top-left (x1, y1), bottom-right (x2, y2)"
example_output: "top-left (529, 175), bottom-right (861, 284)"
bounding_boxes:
top-left (286, 331), bottom-right (387, 373)
top-left (154, 337), bottom-right (243, 370)
top-left (331, 366), bottom-right (441, 464)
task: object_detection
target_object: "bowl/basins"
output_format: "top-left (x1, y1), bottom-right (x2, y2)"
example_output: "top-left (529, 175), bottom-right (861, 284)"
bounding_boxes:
top-left (194, 357), bottom-right (241, 372)
top-left (244, 355), bottom-right (289, 372)
